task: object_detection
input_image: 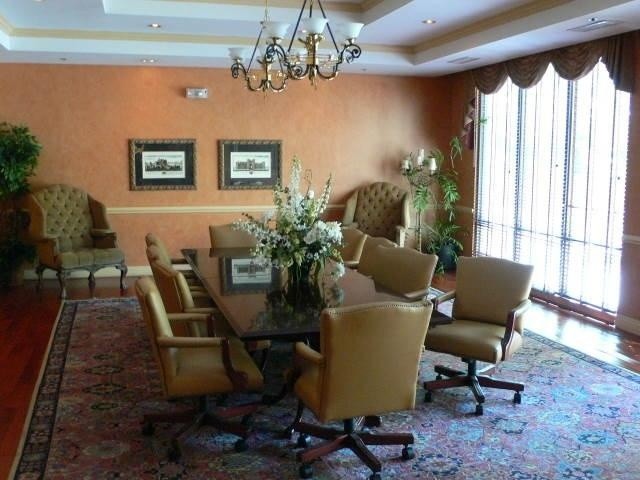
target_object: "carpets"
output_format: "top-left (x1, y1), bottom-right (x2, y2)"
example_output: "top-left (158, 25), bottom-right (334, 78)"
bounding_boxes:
top-left (9, 295), bottom-right (640, 480)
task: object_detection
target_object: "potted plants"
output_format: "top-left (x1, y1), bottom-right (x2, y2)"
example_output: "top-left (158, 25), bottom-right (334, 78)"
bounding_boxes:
top-left (0, 121), bottom-right (43, 288)
top-left (405, 137), bottom-right (469, 275)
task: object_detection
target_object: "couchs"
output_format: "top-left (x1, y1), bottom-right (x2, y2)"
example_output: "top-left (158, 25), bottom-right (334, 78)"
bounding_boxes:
top-left (424, 256), bottom-right (533, 415)
top-left (146, 244), bottom-right (213, 306)
top-left (145, 233), bottom-right (198, 278)
top-left (135, 278), bottom-right (264, 459)
top-left (30, 184), bottom-right (128, 301)
top-left (343, 183), bottom-right (410, 248)
top-left (150, 259), bottom-right (271, 407)
top-left (336, 228), bottom-right (367, 270)
top-left (356, 237), bottom-right (399, 291)
top-left (377, 246), bottom-right (439, 301)
top-left (209, 224), bottom-right (261, 249)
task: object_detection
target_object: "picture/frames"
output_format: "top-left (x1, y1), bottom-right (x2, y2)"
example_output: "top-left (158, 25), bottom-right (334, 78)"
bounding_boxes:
top-left (218, 139), bottom-right (283, 191)
top-left (220, 257), bottom-right (271, 294)
top-left (129, 138), bottom-right (197, 192)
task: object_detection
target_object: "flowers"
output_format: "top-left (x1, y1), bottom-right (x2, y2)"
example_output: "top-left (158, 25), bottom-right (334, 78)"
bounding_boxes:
top-left (229, 155), bottom-right (347, 304)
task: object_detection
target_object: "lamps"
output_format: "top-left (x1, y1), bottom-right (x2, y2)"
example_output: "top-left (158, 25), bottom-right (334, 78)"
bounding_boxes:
top-left (264, 0), bottom-right (364, 89)
top-left (400, 149), bottom-right (437, 249)
top-left (229, 0), bottom-right (305, 102)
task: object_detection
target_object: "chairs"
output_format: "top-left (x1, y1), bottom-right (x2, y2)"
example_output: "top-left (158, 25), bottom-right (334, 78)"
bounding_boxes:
top-left (266, 303), bottom-right (434, 480)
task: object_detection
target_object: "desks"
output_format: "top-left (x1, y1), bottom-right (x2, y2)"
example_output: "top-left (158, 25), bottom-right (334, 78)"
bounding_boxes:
top-left (181, 248), bottom-right (455, 426)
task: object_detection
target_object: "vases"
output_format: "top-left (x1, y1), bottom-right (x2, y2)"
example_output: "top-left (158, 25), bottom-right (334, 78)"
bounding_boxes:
top-left (287, 257), bottom-right (311, 306)
